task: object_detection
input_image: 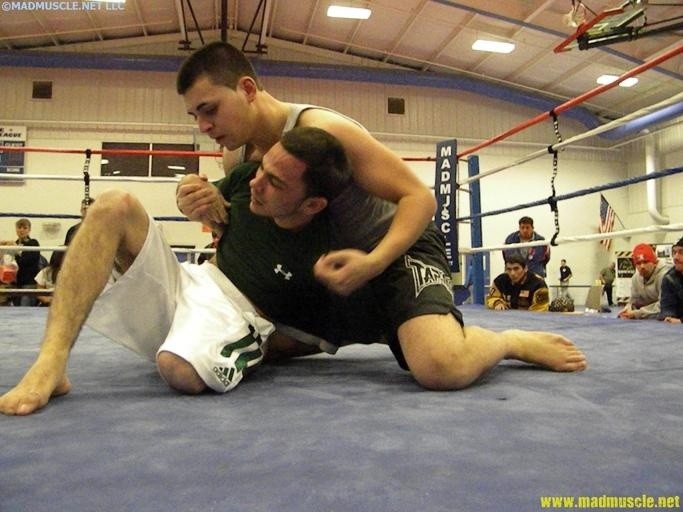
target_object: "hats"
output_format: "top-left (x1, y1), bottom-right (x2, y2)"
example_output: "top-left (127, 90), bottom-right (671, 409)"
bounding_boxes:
top-left (632, 242), bottom-right (658, 268)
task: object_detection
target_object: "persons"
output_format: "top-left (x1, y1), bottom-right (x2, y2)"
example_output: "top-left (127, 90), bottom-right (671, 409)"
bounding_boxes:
top-left (618, 243), bottom-right (671, 319)
top-left (0, 128), bottom-right (353, 416)
top-left (196, 229), bottom-right (221, 265)
top-left (659, 232), bottom-right (682, 322)
top-left (484, 253), bottom-right (549, 312)
top-left (501, 215), bottom-right (551, 278)
top-left (174, 43), bottom-right (590, 388)
top-left (0, 196), bottom-right (97, 307)
top-left (599, 262), bottom-right (616, 306)
top-left (557, 257), bottom-right (572, 300)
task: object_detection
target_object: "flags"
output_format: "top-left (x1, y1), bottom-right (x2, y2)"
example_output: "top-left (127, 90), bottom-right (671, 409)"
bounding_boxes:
top-left (598, 196), bottom-right (614, 250)
